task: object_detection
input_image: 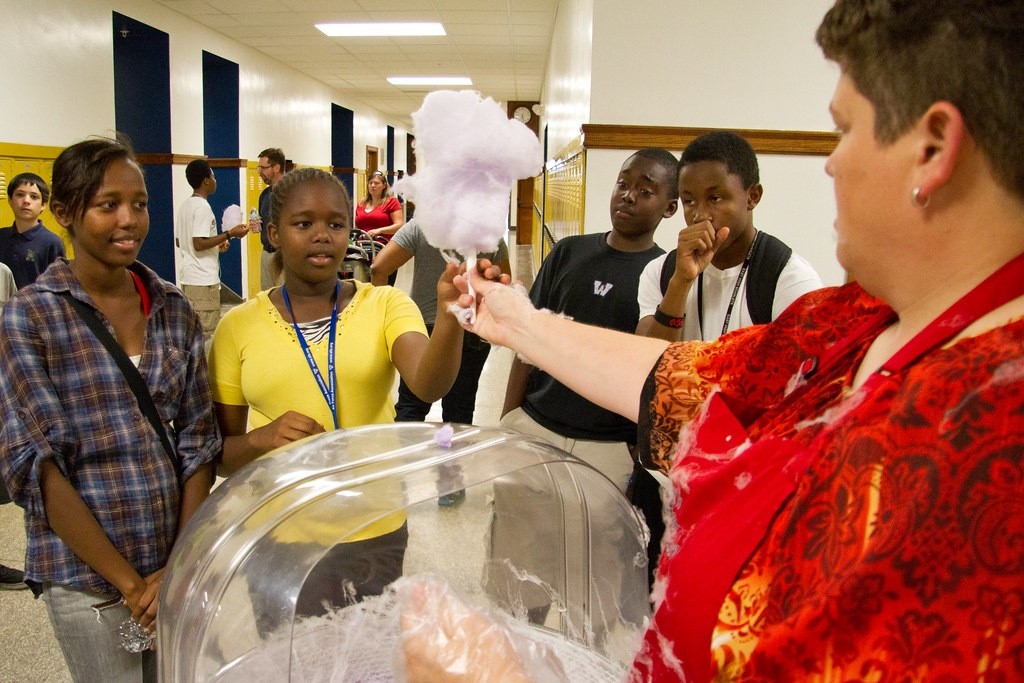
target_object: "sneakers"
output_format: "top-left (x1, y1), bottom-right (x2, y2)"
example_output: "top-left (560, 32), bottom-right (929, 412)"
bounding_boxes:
top-left (0, 563), bottom-right (29, 590)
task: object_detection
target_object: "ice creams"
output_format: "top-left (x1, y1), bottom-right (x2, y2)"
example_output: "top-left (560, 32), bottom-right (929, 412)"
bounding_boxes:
top-left (250, 209), bottom-right (260, 233)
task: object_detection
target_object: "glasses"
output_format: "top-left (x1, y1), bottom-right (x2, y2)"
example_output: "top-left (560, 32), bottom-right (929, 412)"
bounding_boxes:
top-left (257, 163), bottom-right (277, 170)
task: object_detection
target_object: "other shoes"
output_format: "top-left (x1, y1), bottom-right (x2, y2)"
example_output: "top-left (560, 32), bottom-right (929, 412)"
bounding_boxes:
top-left (438, 463), bottom-right (465, 506)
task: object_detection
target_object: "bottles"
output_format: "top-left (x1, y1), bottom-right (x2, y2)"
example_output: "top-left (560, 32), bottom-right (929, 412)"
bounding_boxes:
top-left (120, 617), bottom-right (153, 654)
top-left (250, 207), bottom-right (260, 233)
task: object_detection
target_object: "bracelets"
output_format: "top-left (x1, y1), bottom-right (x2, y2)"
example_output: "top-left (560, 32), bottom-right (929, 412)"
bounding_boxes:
top-left (654, 305), bottom-right (687, 329)
top-left (223, 230), bottom-right (231, 241)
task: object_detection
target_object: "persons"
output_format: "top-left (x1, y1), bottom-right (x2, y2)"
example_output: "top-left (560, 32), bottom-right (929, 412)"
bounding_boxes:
top-left (0, 132), bottom-right (824, 681)
top-left (444, 0), bottom-right (1023, 682)
